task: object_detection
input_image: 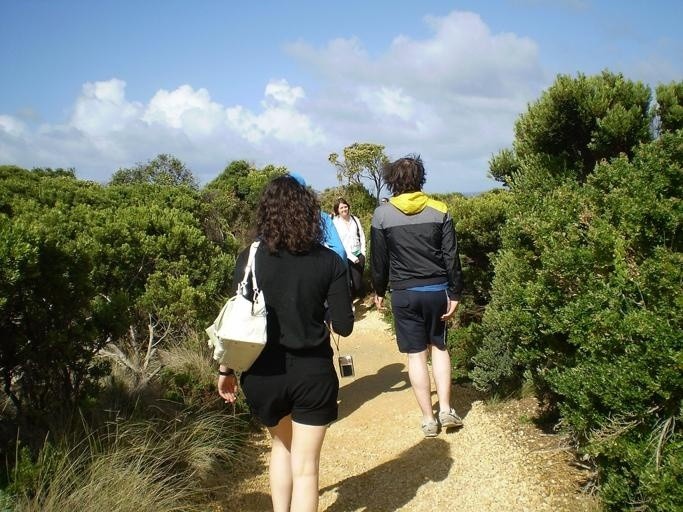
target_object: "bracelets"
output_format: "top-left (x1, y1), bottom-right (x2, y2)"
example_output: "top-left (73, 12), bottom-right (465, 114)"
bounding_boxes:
top-left (219, 369), bottom-right (233, 375)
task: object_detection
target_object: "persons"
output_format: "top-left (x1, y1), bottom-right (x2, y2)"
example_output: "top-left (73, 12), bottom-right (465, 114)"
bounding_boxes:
top-left (370, 154), bottom-right (463, 437)
top-left (291, 172), bottom-right (366, 301)
top-left (217, 176), bottom-right (355, 512)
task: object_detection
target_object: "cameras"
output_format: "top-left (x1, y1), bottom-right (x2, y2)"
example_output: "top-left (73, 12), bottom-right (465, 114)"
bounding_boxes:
top-left (338, 355), bottom-right (355, 378)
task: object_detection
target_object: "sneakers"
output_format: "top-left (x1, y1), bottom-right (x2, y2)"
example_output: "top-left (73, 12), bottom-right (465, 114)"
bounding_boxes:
top-left (421, 409), bottom-right (463, 437)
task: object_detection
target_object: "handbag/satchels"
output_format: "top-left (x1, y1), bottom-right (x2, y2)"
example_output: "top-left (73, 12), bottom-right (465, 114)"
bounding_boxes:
top-left (205, 290), bottom-right (268, 372)
